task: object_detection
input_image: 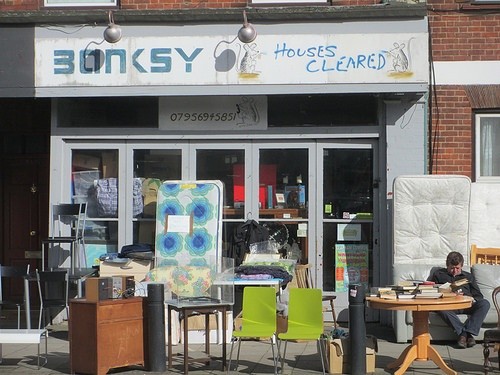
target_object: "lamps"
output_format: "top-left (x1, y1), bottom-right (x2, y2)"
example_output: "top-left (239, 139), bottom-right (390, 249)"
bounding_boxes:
top-left (103, 10), bottom-right (122, 43)
top-left (238, 9), bottom-right (257, 43)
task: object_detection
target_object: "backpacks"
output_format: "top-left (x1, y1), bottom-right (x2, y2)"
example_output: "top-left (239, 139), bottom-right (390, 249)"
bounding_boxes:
top-left (121, 242), bottom-right (153, 253)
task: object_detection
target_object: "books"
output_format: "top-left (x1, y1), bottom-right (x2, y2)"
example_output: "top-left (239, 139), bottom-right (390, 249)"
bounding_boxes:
top-left (369, 273), bottom-right (457, 300)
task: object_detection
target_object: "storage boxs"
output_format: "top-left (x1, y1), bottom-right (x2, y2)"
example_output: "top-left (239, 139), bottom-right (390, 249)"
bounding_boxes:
top-left (99, 260), bottom-right (154, 284)
top-left (321, 335), bottom-right (378, 374)
top-left (84, 276), bottom-right (127, 299)
top-left (234, 310), bottom-right (270, 342)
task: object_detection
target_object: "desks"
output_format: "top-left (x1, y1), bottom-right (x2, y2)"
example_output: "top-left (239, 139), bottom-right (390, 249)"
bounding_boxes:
top-left (68, 298), bottom-right (143, 375)
top-left (167, 304), bottom-right (228, 375)
top-left (0, 329), bottom-right (47, 370)
top-left (213, 274), bottom-right (284, 302)
top-left (23, 268), bottom-right (100, 329)
top-left (365, 291), bottom-right (472, 375)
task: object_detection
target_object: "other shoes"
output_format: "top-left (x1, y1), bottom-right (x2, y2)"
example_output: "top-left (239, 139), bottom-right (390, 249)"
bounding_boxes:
top-left (456, 333), bottom-right (467, 348)
top-left (467, 337), bottom-right (476, 347)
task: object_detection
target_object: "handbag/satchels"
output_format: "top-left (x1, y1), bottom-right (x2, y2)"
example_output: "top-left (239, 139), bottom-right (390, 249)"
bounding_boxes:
top-left (92, 176), bottom-right (144, 217)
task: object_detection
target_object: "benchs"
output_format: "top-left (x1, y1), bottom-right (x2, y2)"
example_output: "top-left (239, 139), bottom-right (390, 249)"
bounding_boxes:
top-left (391, 245), bottom-right (500, 375)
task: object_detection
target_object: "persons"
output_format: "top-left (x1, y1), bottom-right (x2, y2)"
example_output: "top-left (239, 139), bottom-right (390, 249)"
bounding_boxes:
top-left (433, 251), bottom-right (490, 348)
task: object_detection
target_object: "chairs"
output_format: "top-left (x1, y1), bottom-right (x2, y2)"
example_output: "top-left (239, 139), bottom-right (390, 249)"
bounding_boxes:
top-left (295, 263), bottom-right (338, 329)
top-left (227, 286), bottom-right (282, 375)
top-left (278, 288), bottom-right (327, 375)
top-left (35, 201), bottom-right (88, 328)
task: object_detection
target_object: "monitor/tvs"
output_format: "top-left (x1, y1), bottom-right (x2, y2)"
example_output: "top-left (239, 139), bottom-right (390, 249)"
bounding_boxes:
top-left (284, 186), bottom-right (305, 206)
